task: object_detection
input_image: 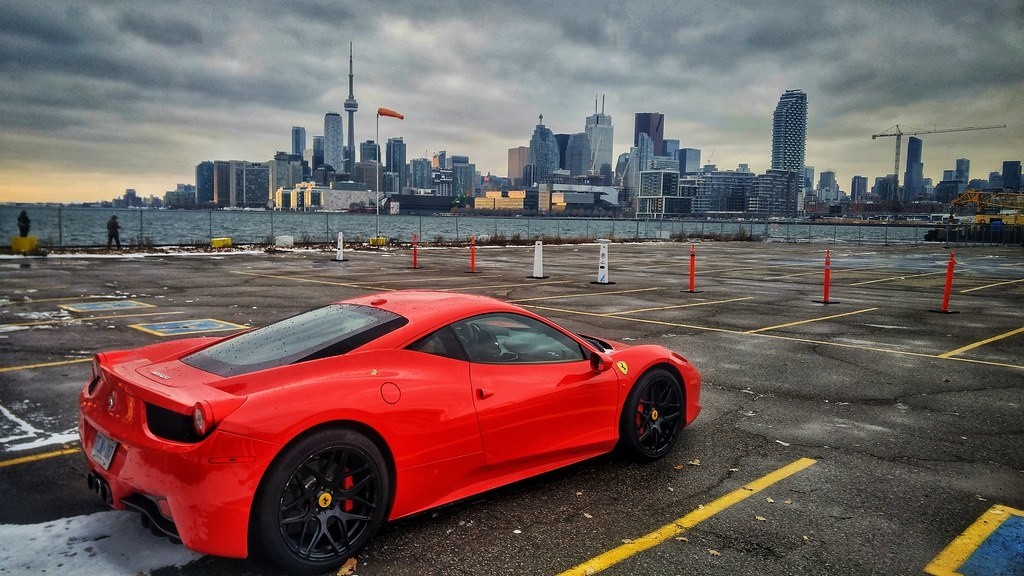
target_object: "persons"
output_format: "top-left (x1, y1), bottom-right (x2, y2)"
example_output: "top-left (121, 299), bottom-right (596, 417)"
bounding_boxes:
top-left (17, 210), bottom-right (31, 237)
top-left (107, 216), bottom-right (123, 250)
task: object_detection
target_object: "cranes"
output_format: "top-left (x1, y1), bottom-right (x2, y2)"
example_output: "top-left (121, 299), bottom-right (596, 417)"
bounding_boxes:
top-left (871, 123), bottom-right (1007, 211)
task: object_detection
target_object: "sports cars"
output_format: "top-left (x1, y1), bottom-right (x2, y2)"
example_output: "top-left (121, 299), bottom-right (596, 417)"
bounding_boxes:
top-left (79, 291), bottom-right (702, 572)
